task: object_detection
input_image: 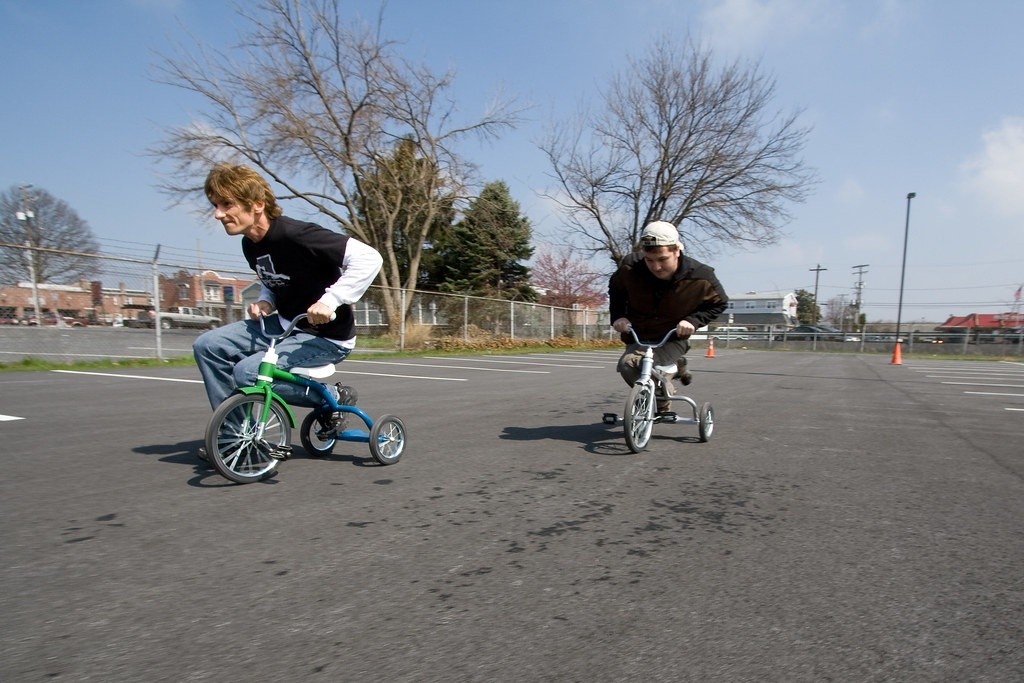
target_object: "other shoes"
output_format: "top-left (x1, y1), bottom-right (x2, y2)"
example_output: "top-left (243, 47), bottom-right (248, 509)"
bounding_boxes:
top-left (676, 357), bottom-right (692, 386)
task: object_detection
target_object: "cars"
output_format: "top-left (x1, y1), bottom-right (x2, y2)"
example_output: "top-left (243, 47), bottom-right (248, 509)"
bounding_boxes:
top-left (0, 311), bottom-right (107, 327)
top-left (774, 324), bottom-right (904, 342)
top-left (920, 337), bottom-right (944, 344)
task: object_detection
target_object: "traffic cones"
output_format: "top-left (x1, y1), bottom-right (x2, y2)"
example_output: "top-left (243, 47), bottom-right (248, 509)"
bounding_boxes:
top-left (888, 343), bottom-right (903, 367)
top-left (704, 337), bottom-right (717, 358)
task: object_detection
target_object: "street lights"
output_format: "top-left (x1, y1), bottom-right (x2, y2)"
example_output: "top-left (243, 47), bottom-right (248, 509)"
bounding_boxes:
top-left (895, 190), bottom-right (917, 342)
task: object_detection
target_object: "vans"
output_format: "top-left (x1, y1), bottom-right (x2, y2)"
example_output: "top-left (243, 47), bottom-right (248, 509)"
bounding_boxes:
top-left (710, 327), bottom-right (750, 341)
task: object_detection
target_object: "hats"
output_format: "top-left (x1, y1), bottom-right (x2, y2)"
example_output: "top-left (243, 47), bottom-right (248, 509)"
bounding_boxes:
top-left (642, 220), bottom-right (684, 250)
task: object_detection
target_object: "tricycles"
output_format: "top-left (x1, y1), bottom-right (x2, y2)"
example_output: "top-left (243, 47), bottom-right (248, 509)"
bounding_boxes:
top-left (603, 327), bottom-right (715, 455)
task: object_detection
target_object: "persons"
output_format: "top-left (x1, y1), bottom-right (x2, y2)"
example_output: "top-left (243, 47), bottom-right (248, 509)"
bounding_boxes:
top-left (192, 161), bottom-right (383, 463)
top-left (609, 219), bottom-right (729, 410)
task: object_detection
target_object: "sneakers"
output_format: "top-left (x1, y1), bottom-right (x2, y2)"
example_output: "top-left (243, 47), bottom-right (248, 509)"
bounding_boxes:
top-left (197, 437), bottom-right (264, 466)
top-left (316, 382), bottom-right (359, 442)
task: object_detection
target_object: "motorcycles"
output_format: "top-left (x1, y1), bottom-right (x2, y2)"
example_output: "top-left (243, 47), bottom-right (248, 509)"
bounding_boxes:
top-left (205, 306), bottom-right (408, 482)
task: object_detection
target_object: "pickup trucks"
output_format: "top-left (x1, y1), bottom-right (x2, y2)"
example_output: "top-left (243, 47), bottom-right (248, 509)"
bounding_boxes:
top-left (138, 306), bottom-right (223, 330)
